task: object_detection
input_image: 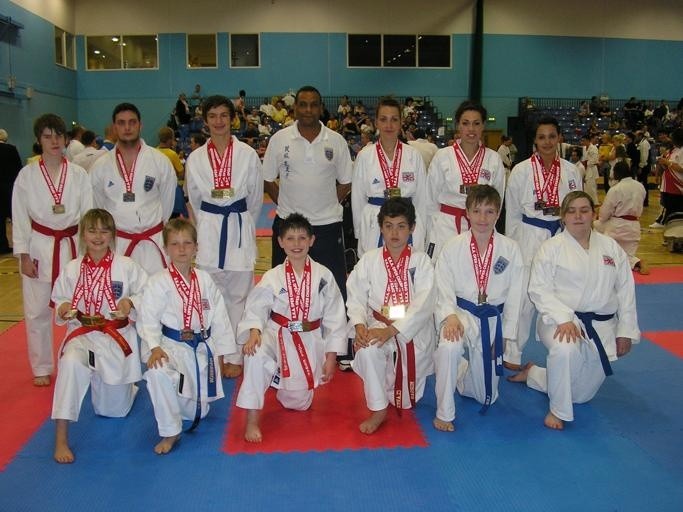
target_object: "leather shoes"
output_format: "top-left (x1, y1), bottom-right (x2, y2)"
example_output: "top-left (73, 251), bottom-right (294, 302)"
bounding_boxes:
top-left (0, 246), bottom-right (13, 254)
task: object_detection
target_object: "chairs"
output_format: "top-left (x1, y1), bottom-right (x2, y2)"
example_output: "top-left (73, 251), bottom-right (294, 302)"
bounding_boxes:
top-left (541, 106), bottom-right (625, 144)
top-left (175, 97), bottom-right (448, 159)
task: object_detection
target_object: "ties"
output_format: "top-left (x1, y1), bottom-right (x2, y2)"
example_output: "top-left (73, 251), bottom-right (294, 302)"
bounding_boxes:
top-left (560, 143), bottom-right (563, 158)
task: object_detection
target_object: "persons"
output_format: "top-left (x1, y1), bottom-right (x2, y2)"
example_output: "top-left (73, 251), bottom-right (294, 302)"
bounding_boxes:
top-left (0, 84), bottom-right (682, 462)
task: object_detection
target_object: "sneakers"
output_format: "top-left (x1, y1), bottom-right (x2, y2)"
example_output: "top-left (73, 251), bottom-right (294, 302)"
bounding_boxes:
top-left (648, 222), bottom-right (666, 229)
top-left (338, 360), bottom-right (354, 372)
